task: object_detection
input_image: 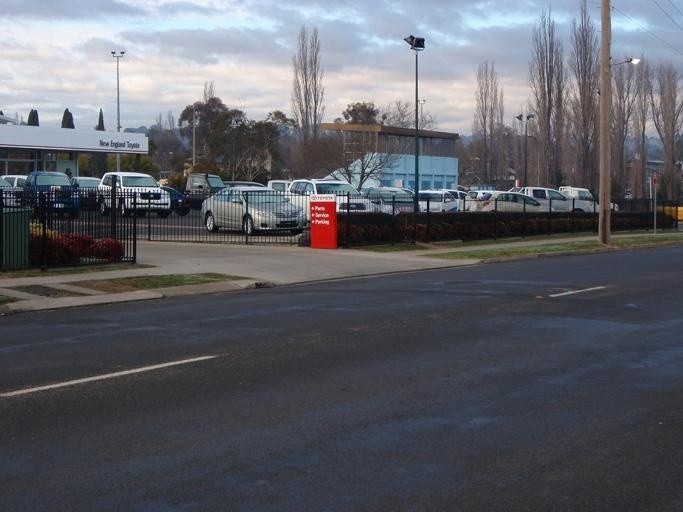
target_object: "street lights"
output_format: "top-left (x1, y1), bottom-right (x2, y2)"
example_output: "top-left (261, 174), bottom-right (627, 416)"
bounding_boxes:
top-left (517, 112), bottom-right (536, 186)
top-left (403, 34), bottom-right (425, 244)
